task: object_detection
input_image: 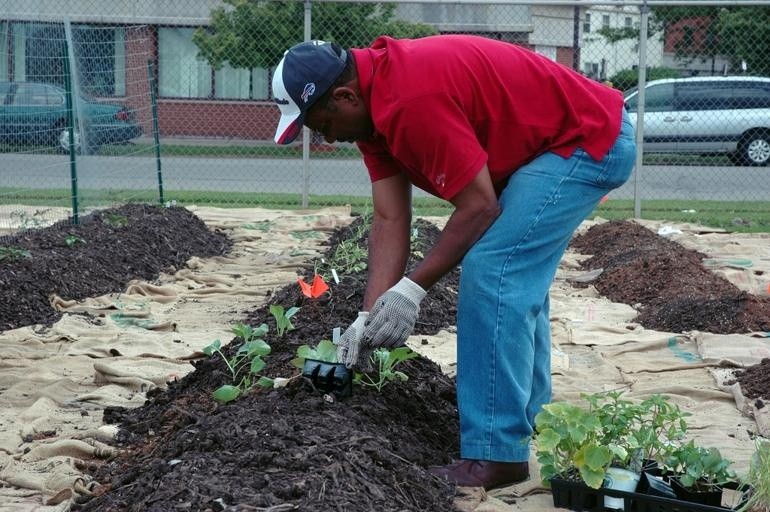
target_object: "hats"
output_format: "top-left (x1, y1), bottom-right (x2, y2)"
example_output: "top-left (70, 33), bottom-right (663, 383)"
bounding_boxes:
top-left (271, 40), bottom-right (347, 145)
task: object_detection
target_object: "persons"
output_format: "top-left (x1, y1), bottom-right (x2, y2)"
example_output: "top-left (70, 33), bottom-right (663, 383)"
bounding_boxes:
top-left (272, 34), bottom-right (636, 493)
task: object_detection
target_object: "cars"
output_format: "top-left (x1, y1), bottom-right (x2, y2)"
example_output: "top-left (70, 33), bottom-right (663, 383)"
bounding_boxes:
top-left (1, 78), bottom-right (146, 153)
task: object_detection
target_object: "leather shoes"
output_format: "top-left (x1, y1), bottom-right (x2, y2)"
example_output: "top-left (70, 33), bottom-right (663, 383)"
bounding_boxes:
top-left (428, 457), bottom-right (528, 491)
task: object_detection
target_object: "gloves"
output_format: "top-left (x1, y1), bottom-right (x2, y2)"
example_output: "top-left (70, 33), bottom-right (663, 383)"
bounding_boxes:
top-left (337, 312), bottom-right (375, 371)
top-left (362, 276), bottom-right (427, 350)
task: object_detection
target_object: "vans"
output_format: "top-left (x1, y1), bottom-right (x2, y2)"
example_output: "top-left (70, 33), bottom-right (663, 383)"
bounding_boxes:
top-left (604, 73), bottom-right (769, 166)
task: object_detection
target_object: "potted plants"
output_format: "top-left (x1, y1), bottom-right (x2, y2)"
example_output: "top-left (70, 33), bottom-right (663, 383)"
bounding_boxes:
top-left (290, 340), bottom-right (419, 401)
top-left (660, 440), bottom-right (739, 506)
top-left (520, 389), bottom-right (692, 512)
